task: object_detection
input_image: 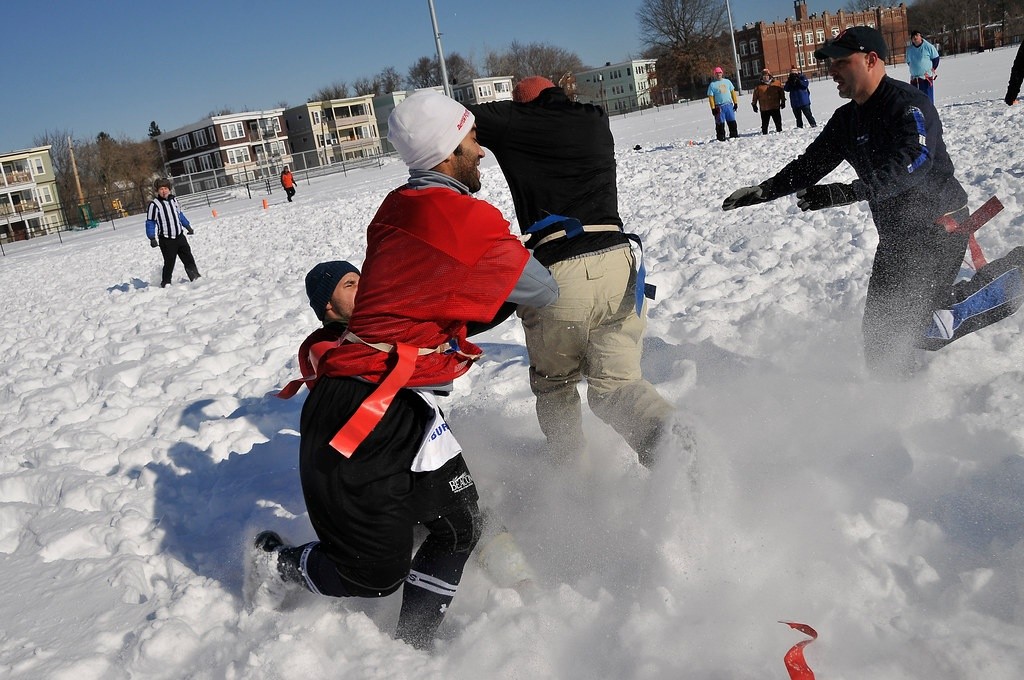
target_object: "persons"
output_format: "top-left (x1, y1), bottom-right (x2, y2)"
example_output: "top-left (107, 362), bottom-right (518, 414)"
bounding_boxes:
top-left (905, 30), bottom-right (940, 105)
top-left (707, 66), bottom-right (738, 141)
top-left (723, 26), bottom-right (1024, 375)
top-left (146, 178), bottom-right (201, 288)
top-left (1004, 38), bottom-right (1024, 106)
top-left (751, 69), bottom-right (787, 134)
top-left (281, 166), bottom-right (297, 202)
top-left (277, 260), bottom-right (360, 404)
top-left (466, 74), bottom-right (697, 472)
top-left (784, 65), bottom-right (817, 129)
top-left (254, 91), bottom-right (556, 651)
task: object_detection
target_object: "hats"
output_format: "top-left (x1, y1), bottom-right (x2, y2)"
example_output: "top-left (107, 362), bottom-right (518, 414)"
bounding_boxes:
top-left (910, 30), bottom-right (922, 36)
top-left (387, 89), bottom-right (476, 171)
top-left (154, 178), bottom-right (173, 190)
top-left (714, 66), bottom-right (723, 76)
top-left (305, 261), bottom-right (360, 320)
top-left (813, 26), bottom-right (890, 66)
top-left (791, 65), bottom-right (797, 69)
top-left (512, 77), bottom-right (556, 102)
top-left (758, 69), bottom-right (774, 82)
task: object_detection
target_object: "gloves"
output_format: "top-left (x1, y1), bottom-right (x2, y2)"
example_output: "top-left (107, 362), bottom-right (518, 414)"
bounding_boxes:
top-left (752, 104), bottom-right (758, 113)
top-left (796, 181), bottom-right (869, 212)
top-left (294, 182), bottom-right (298, 187)
top-left (187, 227), bottom-right (195, 235)
top-left (780, 100), bottom-right (785, 109)
top-left (722, 179), bottom-right (776, 212)
top-left (1004, 90), bottom-right (1019, 107)
top-left (712, 109), bottom-right (718, 115)
top-left (150, 237), bottom-right (157, 248)
top-left (732, 103), bottom-right (738, 111)
top-left (284, 186), bottom-right (286, 191)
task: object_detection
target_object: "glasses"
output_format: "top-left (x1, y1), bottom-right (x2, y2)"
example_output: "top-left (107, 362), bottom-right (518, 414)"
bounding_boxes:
top-left (823, 58), bottom-right (867, 70)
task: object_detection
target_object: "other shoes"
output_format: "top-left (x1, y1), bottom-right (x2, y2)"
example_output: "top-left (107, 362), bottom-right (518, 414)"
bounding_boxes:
top-left (242, 531), bottom-right (287, 615)
top-left (287, 198), bottom-right (292, 202)
